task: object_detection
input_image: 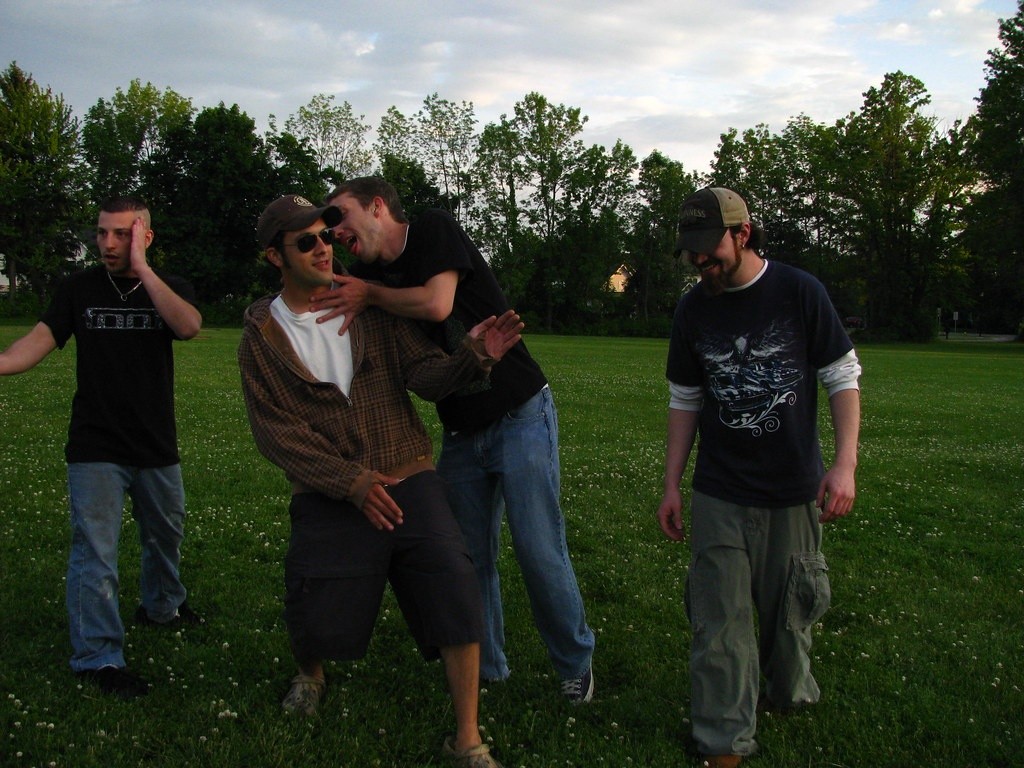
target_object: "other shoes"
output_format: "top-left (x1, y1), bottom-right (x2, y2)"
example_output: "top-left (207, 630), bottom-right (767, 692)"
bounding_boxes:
top-left (80, 669), bottom-right (145, 705)
top-left (140, 602), bottom-right (204, 632)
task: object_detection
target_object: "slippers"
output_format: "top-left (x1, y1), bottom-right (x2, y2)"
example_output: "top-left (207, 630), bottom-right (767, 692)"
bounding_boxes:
top-left (283, 675), bottom-right (324, 715)
top-left (442, 735), bottom-right (498, 768)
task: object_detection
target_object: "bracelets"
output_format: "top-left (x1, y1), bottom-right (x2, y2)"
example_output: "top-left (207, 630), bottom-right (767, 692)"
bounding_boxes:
top-left (366, 282), bottom-right (383, 314)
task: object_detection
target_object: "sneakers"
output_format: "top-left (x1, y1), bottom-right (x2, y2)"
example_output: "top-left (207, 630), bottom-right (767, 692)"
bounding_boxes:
top-left (555, 659), bottom-right (593, 703)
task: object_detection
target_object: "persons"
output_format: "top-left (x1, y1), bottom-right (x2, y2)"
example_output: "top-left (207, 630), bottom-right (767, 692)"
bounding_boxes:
top-left (0, 194), bottom-right (204, 702)
top-left (237, 194), bottom-right (524, 768)
top-left (310, 177), bottom-right (596, 704)
top-left (656, 187), bottom-right (862, 768)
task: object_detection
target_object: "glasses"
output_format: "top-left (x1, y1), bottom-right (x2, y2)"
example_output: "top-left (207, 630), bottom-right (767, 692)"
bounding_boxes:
top-left (274, 226), bottom-right (337, 254)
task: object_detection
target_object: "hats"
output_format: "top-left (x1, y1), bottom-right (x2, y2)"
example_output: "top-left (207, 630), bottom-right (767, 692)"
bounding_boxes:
top-left (257, 195), bottom-right (344, 249)
top-left (672, 186), bottom-right (752, 261)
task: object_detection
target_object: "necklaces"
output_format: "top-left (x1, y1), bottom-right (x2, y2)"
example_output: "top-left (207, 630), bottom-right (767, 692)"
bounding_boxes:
top-left (107, 271), bottom-right (142, 302)
